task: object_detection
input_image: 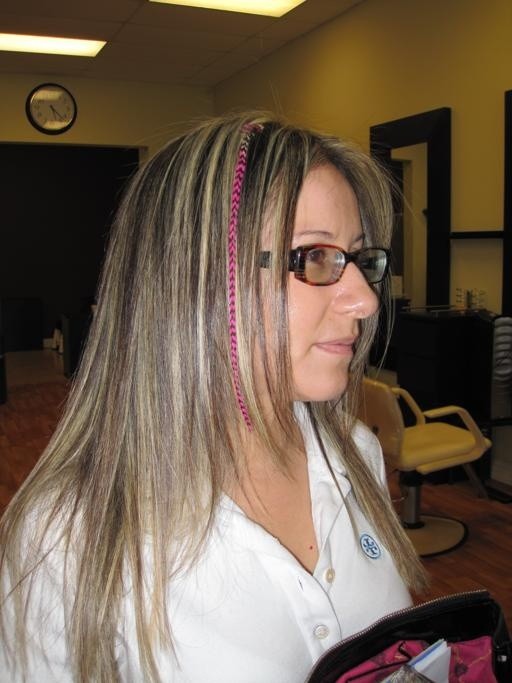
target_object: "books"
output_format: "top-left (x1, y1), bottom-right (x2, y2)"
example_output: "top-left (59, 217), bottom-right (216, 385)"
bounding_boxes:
top-left (381, 638), bottom-right (451, 683)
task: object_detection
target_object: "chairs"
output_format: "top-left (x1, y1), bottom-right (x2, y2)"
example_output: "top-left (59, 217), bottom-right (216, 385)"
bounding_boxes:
top-left (350, 374), bottom-right (492, 556)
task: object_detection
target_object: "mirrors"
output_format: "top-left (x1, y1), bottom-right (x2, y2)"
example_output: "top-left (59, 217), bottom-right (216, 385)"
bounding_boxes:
top-left (370, 107), bottom-right (451, 309)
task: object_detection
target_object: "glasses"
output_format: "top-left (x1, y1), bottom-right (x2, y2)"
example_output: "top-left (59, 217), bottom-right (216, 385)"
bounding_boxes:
top-left (254, 242), bottom-right (393, 288)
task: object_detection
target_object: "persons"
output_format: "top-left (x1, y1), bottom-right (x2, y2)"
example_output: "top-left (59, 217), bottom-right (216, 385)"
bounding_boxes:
top-left (0, 108), bottom-right (431, 683)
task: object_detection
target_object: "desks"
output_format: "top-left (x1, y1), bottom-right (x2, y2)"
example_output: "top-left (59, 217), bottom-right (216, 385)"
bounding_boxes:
top-left (61, 296), bottom-right (95, 379)
top-left (372, 312), bottom-right (493, 484)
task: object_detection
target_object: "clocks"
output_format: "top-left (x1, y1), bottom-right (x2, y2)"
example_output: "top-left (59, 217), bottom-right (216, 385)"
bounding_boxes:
top-left (29, 83), bottom-right (78, 134)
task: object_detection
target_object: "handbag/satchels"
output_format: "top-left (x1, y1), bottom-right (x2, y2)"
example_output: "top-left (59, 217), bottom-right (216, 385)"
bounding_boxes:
top-left (303, 589), bottom-right (511, 683)
